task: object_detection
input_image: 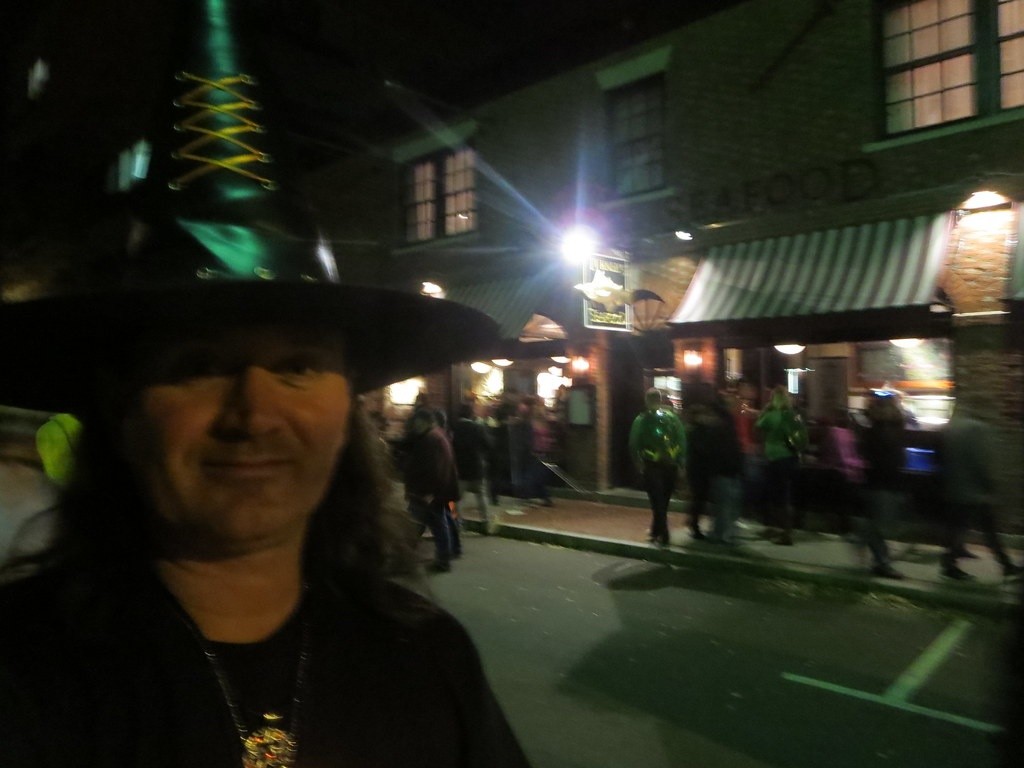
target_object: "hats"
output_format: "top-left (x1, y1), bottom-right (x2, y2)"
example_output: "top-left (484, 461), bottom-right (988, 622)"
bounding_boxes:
top-left (0, 0), bottom-right (498, 414)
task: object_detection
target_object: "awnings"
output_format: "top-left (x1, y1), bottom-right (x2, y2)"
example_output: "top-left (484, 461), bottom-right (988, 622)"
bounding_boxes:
top-left (441, 273), bottom-right (549, 339)
top-left (667, 211), bottom-right (947, 324)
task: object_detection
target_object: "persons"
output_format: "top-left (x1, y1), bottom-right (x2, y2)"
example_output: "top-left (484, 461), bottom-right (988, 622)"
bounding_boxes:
top-left (853, 399), bottom-right (906, 580)
top-left (403, 391), bottom-right (464, 572)
top-left (815, 406), bottom-right (863, 537)
top-left (0, 285), bottom-right (531, 768)
top-left (627, 387), bottom-right (687, 544)
top-left (939, 392), bottom-right (1024, 585)
top-left (448, 392), bottom-right (499, 535)
top-left (752, 384), bottom-right (809, 545)
top-left (685, 379), bottom-right (759, 545)
top-left (487, 394), bottom-right (561, 515)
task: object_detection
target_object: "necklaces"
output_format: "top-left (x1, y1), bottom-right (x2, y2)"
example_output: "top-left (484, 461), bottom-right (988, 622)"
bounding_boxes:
top-left (197, 622), bottom-right (312, 768)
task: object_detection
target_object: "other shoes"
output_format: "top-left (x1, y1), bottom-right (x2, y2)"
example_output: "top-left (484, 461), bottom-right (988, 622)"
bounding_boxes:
top-left (941, 564), bottom-right (969, 579)
top-left (648, 521), bottom-right (671, 546)
top-left (426, 563), bottom-right (449, 572)
top-left (873, 562), bottom-right (900, 579)
top-left (688, 522), bottom-right (706, 539)
top-left (1002, 564), bottom-right (1024, 576)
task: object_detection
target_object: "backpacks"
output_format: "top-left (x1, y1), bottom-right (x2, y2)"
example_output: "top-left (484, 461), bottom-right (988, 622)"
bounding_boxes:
top-left (642, 407), bottom-right (680, 461)
top-left (775, 407), bottom-right (810, 449)
